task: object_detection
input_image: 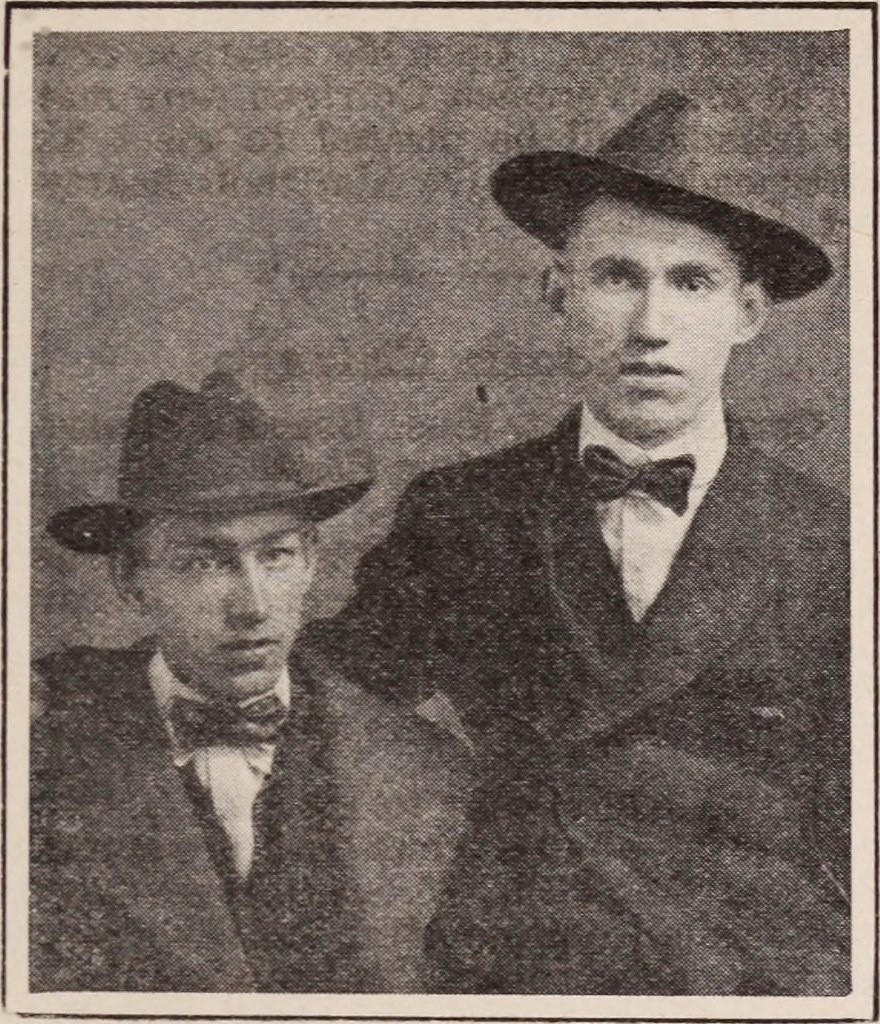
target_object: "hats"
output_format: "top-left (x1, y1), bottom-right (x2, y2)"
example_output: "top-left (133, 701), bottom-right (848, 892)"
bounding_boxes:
top-left (45, 372), bottom-right (374, 556)
top-left (490, 90), bottom-right (833, 302)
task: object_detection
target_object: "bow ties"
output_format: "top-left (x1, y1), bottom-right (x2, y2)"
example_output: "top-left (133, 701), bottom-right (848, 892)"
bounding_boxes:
top-left (169, 696), bottom-right (287, 749)
top-left (580, 444), bottom-right (694, 515)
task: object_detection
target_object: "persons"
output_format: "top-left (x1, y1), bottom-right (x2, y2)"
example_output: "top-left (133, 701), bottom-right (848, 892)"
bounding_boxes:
top-left (28, 372), bottom-right (477, 997)
top-left (30, 88), bottom-right (850, 997)
